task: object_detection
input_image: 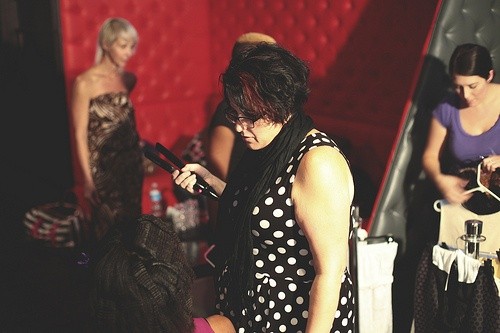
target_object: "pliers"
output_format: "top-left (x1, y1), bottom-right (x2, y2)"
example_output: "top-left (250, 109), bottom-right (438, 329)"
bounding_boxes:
top-left (146, 143), bottom-right (220, 200)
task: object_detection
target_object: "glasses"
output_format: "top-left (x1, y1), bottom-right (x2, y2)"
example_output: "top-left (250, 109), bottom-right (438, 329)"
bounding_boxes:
top-left (223, 108), bottom-right (267, 128)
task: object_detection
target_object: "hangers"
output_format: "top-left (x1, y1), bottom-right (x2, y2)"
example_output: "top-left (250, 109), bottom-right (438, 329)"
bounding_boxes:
top-left (434, 161), bottom-right (500, 212)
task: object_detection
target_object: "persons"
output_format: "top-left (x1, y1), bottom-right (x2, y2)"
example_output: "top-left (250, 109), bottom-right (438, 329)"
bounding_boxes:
top-left (168, 41), bottom-right (356, 333)
top-left (69, 17), bottom-right (143, 245)
top-left (421, 42), bottom-right (500, 204)
top-left (208, 32), bottom-right (279, 263)
top-left (73, 214), bottom-right (237, 333)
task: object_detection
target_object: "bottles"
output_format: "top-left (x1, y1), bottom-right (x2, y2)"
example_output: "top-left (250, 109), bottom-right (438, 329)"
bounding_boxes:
top-left (149, 183), bottom-right (161, 217)
